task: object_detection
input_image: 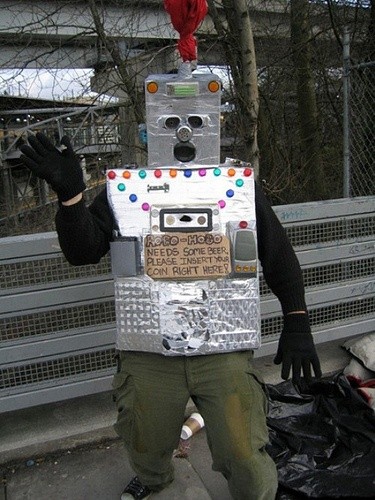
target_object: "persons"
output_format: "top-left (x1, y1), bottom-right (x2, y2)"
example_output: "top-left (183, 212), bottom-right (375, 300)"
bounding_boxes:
top-left (13, 134), bottom-right (25, 149)
top-left (17, 60), bottom-right (323, 500)
top-left (53, 129), bottom-right (60, 148)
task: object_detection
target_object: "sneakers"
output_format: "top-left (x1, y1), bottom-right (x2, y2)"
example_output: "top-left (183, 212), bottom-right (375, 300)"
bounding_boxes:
top-left (120, 476), bottom-right (152, 500)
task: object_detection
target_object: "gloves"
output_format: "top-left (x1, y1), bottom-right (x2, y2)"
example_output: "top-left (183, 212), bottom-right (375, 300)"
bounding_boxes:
top-left (273, 312), bottom-right (322, 385)
top-left (19, 132), bottom-right (86, 202)
